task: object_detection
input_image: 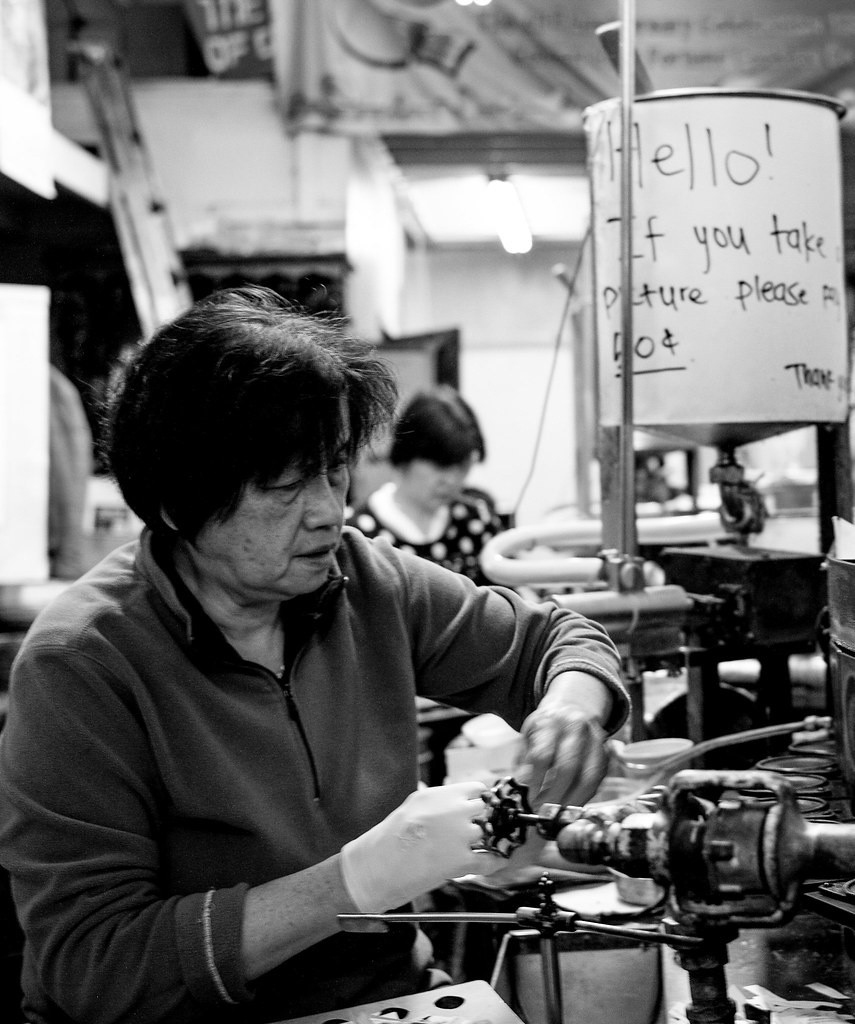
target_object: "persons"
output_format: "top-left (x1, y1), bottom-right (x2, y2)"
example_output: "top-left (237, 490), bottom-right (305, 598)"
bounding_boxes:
top-left (0, 287), bottom-right (632, 1024)
top-left (49, 361), bottom-right (90, 581)
top-left (345, 385), bottom-right (499, 583)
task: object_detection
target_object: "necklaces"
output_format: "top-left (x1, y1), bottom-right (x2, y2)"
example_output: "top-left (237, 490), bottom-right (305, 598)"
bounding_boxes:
top-left (276, 664), bottom-right (286, 678)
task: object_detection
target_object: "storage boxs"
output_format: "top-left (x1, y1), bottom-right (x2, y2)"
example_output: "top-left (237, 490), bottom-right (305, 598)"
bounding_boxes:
top-left (660, 542), bottom-right (828, 647)
